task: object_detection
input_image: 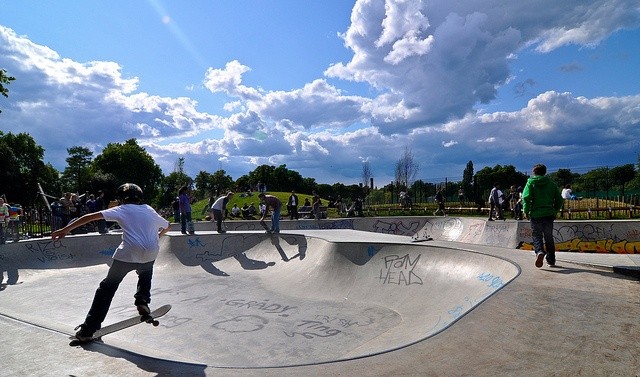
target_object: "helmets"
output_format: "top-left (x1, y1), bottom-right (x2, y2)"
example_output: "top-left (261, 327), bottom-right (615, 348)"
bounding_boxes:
top-left (115, 182), bottom-right (143, 199)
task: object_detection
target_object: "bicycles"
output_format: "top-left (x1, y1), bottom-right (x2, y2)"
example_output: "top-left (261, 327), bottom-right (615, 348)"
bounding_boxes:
top-left (3, 214), bottom-right (23, 242)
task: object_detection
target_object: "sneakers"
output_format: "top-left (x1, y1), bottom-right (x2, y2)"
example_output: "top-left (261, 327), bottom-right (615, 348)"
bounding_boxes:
top-left (137, 305), bottom-right (150, 314)
top-left (535, 251), bottom-right (544, 267)
top-left (75, 326), bottom-right (92, 341)
top-left (548, 263), bottom-right (555, 267)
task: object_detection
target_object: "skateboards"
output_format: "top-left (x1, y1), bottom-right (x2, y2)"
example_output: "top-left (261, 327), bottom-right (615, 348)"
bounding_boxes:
top-left (223, 219), bottom-right (228, 233)
top-left (188, 221), bottom-right (194, 235)
top-left (76, 304), bottom-right (171, 342)
top-left (261, 221), bottom-right (271, 233)
top-left (412, 236), bottom-right (433, 242)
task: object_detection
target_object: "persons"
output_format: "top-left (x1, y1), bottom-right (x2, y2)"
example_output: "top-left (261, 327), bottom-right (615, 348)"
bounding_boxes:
top-left (312, 199), bottom-right (322, 220)
top-left (246, 203), bottom-right (256, 215)
top-left (288, 190), bottom-right (298, 219)
top-left (59, 192), bottom-right (74, 228)
top-left (258, 193), bottom-right (283, 234)
top-left (240, 203), bottom-right (249, 218)
top-left (51, 183), bottom-right (171, 342)
top-left (515, 186), bottom-right (527, 220)
top-left (50, 199), bottom-right (62, 232)
top-left (171, 197), bottom-right (180, 223)
top-left (211, 192), bottom-right (234, 233)
top-left (96, 190), bottom-right (107, 233)
top-left (561, 184), bottom-right (578, 201)
top-left (522, 163), bottom-right (564, 268)
top-left (488, 183), bottom-right (506, 221)
top-left (334, 195), bottom-right (344, 215)
top-left (208, 193), bottom-right (215, 222)
top-left (304, 198), bottom-right (310, 206)
top-left (7, 204), bottom-right (23, 241)
top-left (458, 185), bottom-right (465, 206)
top-left (86, 194), bottom-right (96, 228)
top-left (312, 190), bottom-right (320, 220)
top-left (433, 188), bottom-right (449, 217)
top-left (230, 203), bottom-right (241, 218)
top-left (259, 175), bottom-right (265, 193)
top-left (0, 198), bottom-right (11, 240)
top-left (70, 194), bottom-right (80, 234)
top-left (505, 186), bottom-right (518, 220)
top-left (259, 203), bottom-right (266, 217)
top-left (495, 185), bottom-right (506, 221)
top-left (179, 186), bottom-right (192, 234)
